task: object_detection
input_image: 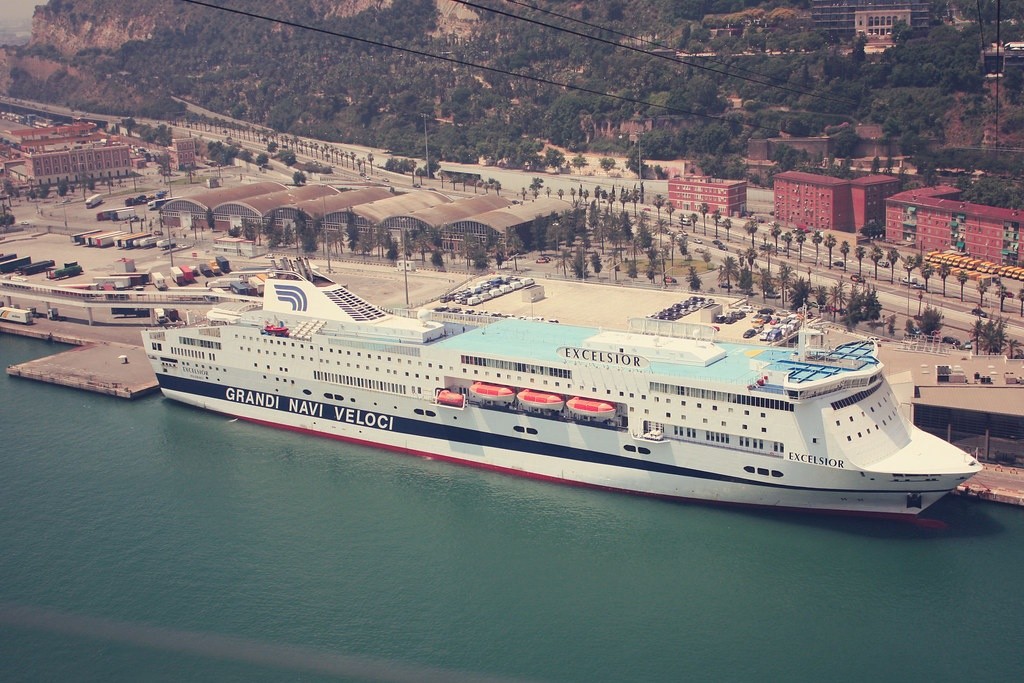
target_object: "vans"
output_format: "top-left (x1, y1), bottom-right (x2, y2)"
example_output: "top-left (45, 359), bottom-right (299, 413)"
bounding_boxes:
top-left (432, 274), bottom-right (559, 323)
top-left (679, 218), bottom-right (692, 226)
top-left (902, 276), bottom-right (918, 284)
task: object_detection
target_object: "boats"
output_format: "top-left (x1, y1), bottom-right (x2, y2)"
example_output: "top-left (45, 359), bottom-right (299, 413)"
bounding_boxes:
top-left (438, 390), bottom-right (464, 406)
top-left (469, 381), bottom-right (516, 403)
top-left (566, 397), bottom-right (616, 419)
top-left (517, 388), bottom-right (566, 412)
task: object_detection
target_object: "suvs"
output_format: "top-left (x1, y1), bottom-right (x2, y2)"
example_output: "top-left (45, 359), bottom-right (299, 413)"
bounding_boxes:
top-left (876, 260), bottom-right (889, 268)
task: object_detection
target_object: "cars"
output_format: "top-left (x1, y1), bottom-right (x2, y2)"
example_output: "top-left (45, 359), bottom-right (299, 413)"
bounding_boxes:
top-left (718, 283), bottom-right (733, 289)
top-left (763, 291), bottom-right (780, 299)
top-left (663, 276), bottom-right (676, 283)
top-left (20, 221), bottom-right (29, 225)
top-left (653, 294), bottom-right (812, 343)
top-left (264, 253), bottom-right (275, 259)
top-left (971, 308), bottom-right (987, 318)
top-left (1000, 292), bottom-right (1014, 298)
top-left (911, 283), bottom-right (926, 289)
top-left (666, 230), bottom-right (728, 251)
top-left (109, 141), bottom-right (156, 164)
top-left (812, 301), bottom-right (839, 313)
top-left (850, 274), bottom-right (866, 283)
top-left (911, 326), bottom-right (973, 349)
top-left (535, 256), bottom-right (550, 264)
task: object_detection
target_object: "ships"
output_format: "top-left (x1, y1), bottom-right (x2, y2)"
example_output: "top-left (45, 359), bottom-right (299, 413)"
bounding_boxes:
top-left (138, 254), bottom-right (984, 525)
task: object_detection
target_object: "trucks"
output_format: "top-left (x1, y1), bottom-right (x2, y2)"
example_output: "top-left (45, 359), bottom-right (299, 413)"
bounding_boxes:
top-left (154, 308), bottom-right (179, 324)
top-left (150, 255), bottom-right (232, 290)
top-left (85, 193), bottom-right (103, 209)
